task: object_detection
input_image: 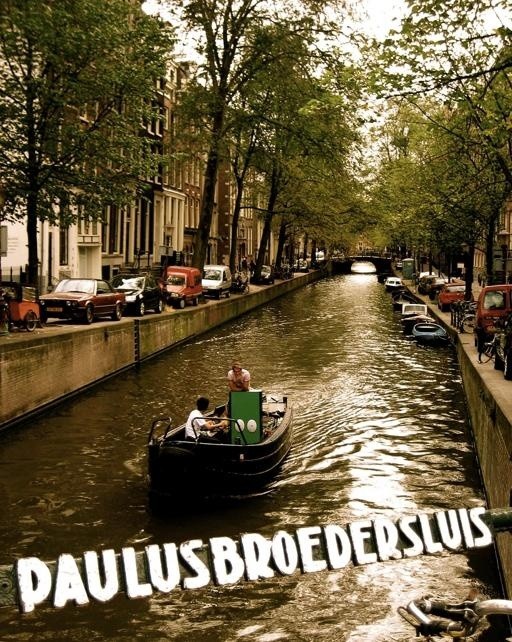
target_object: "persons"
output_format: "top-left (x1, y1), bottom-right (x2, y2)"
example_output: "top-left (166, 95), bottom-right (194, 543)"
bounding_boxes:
top-left (249, 259), bottom-right (256, 276)
top-left (240, 256), bottom-right (248, 275)
top-left (226, 361), bottom-right (252, 393)
top-left (184, 395), bottom-right (228, 442)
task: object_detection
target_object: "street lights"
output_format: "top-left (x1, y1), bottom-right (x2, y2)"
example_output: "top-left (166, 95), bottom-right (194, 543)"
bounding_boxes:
top-left (460, 242), bottom-right (470, 269)
top-left (159, 220), bottom-right (175, 267)
top-left (496, 227), bottom-right (512, 286)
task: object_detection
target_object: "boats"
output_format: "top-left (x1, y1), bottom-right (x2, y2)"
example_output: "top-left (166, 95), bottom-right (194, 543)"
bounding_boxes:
top-left (139, 386), bottom-right (296, 513)
top-left (384, 276), bottom-right (452, 350)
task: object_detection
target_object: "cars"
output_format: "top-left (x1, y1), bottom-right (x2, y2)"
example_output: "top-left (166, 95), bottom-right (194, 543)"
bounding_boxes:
top-left (395, 256), bottom-right (511, 381)
top-left (0, 248), bottom-right (327, 329)
